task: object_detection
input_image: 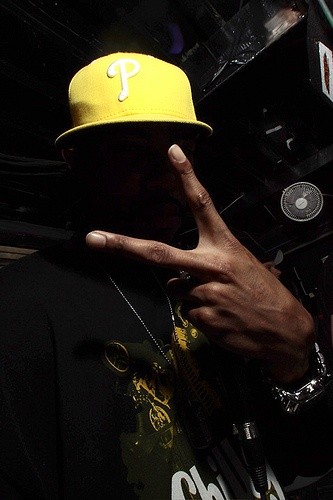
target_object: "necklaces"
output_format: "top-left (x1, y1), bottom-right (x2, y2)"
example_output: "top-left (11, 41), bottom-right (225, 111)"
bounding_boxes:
top-left (98, 258), bottom-right (215, 451)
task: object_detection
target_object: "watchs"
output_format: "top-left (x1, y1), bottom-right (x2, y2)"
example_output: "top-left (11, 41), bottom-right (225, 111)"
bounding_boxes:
top-left (259, 341), bottom-right (333, 415)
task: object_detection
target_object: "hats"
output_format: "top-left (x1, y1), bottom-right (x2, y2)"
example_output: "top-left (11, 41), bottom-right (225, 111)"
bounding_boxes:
top-left (54, 53), bottom-right (214, 141)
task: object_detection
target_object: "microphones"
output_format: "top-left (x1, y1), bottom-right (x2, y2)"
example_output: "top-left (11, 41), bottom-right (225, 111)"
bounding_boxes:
top-left (177, 268), bottom-right (269, 493)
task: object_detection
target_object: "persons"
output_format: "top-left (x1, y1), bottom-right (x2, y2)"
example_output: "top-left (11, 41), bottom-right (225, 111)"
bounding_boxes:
top-left (0, 53), bottom-right (333, 500)
